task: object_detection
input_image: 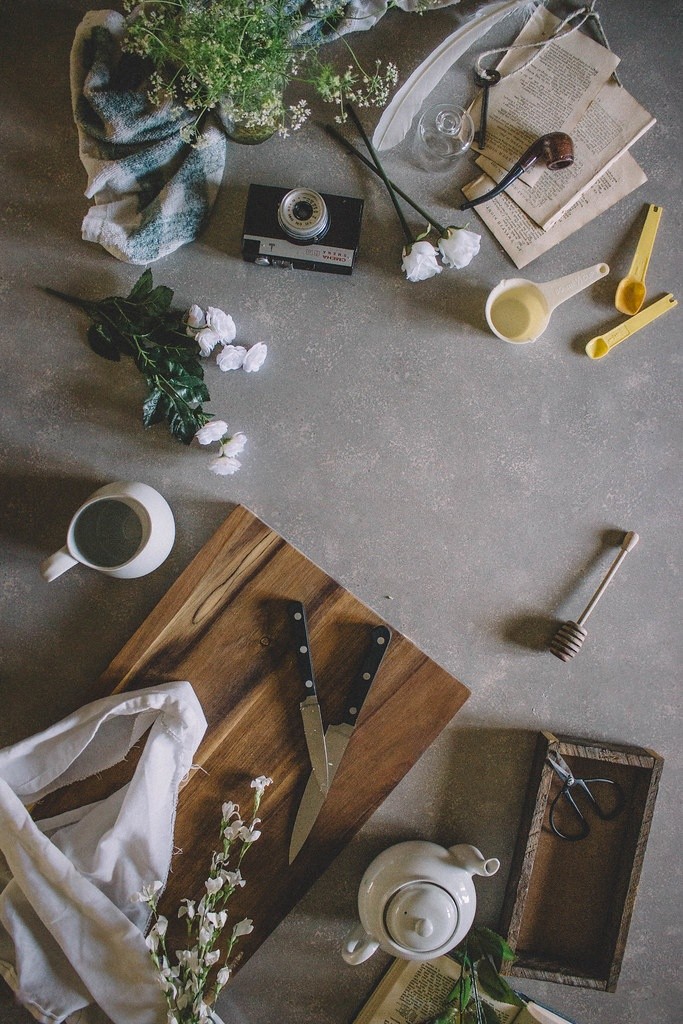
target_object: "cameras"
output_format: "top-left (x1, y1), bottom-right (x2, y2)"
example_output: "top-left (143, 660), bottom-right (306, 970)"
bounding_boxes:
top-left (240, 183), bottom-right (365, 275)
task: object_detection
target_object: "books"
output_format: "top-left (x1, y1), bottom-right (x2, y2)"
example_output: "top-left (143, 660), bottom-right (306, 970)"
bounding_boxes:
top-left (350, 949), bottom-right (580, 1024)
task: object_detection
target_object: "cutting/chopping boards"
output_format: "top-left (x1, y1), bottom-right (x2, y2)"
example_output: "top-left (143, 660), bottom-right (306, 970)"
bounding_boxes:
top-left (0, 503), bottom-right (473, 1011)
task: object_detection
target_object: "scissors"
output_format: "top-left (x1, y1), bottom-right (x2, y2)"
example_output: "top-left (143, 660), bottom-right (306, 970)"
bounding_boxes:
top-left (547, 749), bottom-right (625, 843)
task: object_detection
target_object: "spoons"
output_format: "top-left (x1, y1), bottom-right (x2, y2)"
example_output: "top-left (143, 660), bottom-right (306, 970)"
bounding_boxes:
top-left (584, 293), bottom-right (678, 359)
top-left (614, 205), bottom-right (663, 318)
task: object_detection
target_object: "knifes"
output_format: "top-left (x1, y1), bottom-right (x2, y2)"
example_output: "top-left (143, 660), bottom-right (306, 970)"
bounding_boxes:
top-left (287, 620), bottom-right (393, 865)
top-left (287, 597), bottom-right (330, 803)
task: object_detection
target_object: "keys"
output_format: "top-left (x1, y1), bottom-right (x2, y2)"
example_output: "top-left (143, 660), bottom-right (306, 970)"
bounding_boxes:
top-left (469, 69), bottom-right (503, 150)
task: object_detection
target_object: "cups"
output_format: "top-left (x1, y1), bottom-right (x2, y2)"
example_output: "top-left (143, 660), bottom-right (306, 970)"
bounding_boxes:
top-left (414, 101), bottom-right (475, 174)
top-left (43, 481), bottom-right (176, 584)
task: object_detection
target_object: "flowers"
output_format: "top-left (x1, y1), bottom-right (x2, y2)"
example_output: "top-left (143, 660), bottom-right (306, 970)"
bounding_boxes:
top-left (319, 103), bottom-right (482, 284)
top-left (41, 267), bottom-right (269, 474)
top-left (133, 775), bottom-right (276, 1024)
top-left (124, 2), bottom-right (419, 141)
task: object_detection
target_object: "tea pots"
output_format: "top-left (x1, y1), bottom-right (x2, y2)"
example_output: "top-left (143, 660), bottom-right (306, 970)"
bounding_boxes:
top-left (339, 838), bottom-right (500, 969)
